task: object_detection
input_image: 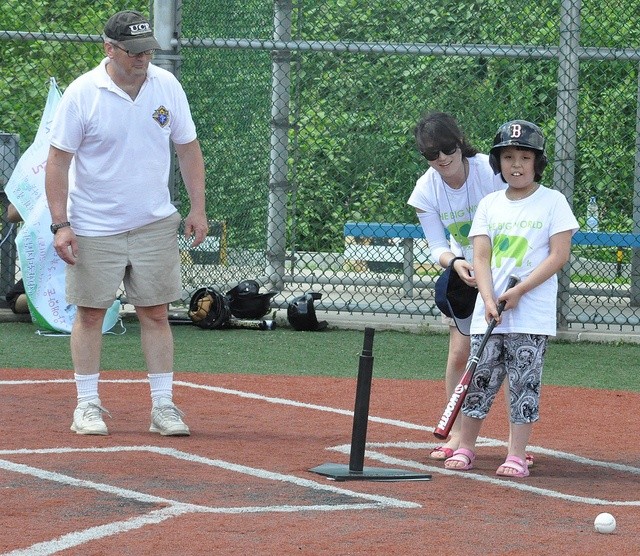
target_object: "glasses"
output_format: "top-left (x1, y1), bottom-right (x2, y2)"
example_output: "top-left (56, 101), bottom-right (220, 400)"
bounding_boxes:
top-left (421, 140), bottom-right (459, 161)
top-left (109, 44), bottom-right (156, 58)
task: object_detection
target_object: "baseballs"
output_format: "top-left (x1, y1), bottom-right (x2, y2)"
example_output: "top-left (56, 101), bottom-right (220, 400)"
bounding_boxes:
top-left (594, 512), bottom-right (617, 534)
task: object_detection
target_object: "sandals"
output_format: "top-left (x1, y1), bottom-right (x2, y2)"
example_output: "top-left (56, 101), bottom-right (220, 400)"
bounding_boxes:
top-left (496, 455), bottom-right (529, 477)
top-left (444, 447), bottom-right (476, 470)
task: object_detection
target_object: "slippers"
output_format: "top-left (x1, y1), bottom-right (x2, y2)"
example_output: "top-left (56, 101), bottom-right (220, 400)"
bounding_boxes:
top-left (524, 453), bottom-right (534, 468)
top-left (429, 446), bottom-right (454, 460)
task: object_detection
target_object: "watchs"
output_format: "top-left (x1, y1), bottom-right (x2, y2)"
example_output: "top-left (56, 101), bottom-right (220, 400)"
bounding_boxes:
top-left (51, 221), bottom-right (71, 234)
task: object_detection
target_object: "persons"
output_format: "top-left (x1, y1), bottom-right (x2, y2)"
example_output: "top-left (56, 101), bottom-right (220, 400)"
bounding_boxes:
top-left (7, 201), bottom-right (32, 314)
top-left (444, 119), bottom-right (581, 478)
top-left (47, 13), bottom-right (210, 438)
top-left (406, 113), bottom-right (536, 469)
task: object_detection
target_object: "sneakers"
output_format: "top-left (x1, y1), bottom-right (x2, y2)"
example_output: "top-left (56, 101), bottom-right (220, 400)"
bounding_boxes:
top-left (69, 397), bottom-right (110, 435)
top-left (149, 397), bottom-right (190, 436)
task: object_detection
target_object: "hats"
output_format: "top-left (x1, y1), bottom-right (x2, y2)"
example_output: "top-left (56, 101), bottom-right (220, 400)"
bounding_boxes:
top-left (103, 10), bottom-right (163, 53)
top-left (434, 256), bottom-right (478, 337)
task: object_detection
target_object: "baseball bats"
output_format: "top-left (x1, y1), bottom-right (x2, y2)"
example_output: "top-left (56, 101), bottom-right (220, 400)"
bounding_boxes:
top-left (433, 274), bottom-right (521, 439)
top-left (166, 320), bottom-right (267, 330)
top-left (168, 314), bottom-right (275, 330)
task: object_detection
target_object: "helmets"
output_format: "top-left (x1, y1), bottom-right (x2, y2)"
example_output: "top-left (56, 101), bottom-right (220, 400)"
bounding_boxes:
top-left (489, 119), bottom-right (547, 178)
top-left (226, 280), bottom-right (278, 320)
top-left (188, 286), bottom-right (231, 330)
top-left (286, 293), bottom-right (329, 332)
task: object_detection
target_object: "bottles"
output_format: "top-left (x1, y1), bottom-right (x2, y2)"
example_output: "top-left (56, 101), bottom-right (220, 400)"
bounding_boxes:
top-left (586, 197), bottom-right (599, 233)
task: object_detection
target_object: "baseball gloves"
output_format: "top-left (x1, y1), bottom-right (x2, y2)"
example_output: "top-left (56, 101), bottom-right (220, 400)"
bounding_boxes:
top-left (188, 295), bottom-right (216, 322)
